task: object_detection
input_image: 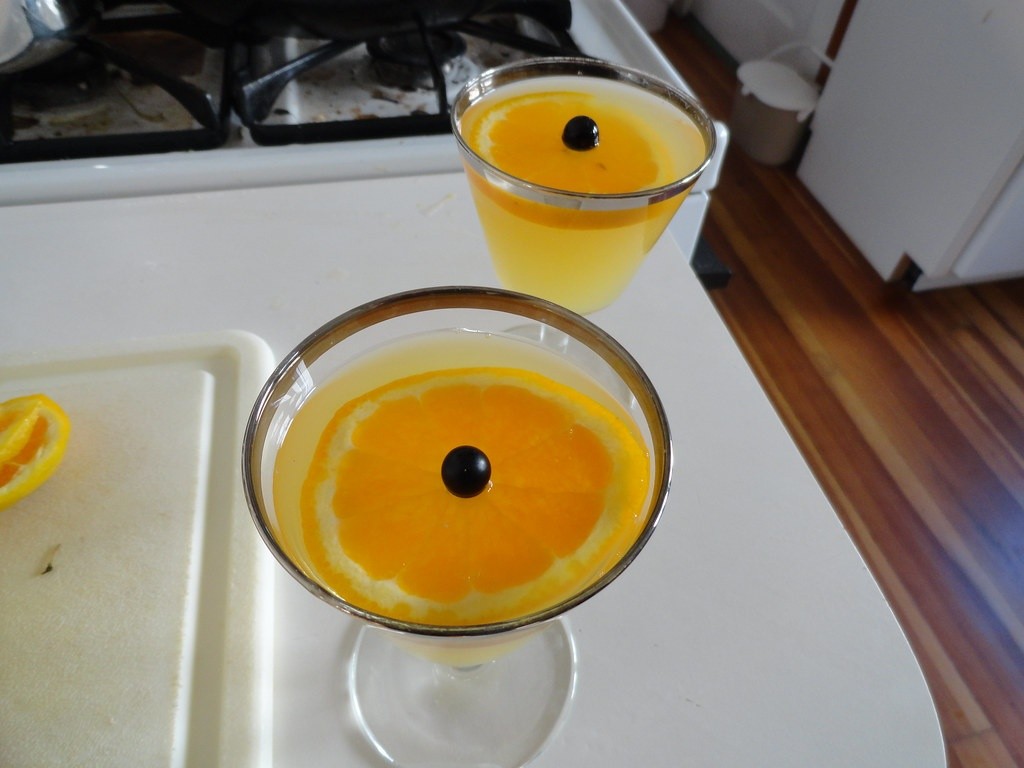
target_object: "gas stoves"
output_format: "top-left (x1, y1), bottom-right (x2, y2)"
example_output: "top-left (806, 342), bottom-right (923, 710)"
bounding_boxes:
top-left (0, 0), bottom-right (585, 207)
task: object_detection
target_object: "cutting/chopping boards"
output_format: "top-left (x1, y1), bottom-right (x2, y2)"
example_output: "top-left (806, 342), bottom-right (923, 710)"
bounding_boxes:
top-left (2, 326), bottom-right (278, 768)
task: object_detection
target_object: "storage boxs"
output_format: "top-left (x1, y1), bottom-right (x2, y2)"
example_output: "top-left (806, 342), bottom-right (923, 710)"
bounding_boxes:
top-left (733, 42), bottom-right (833, 168)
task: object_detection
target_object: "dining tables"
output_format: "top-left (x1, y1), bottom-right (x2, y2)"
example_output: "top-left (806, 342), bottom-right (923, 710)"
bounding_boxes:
top-left (1, 160), bottom-right (948, 768)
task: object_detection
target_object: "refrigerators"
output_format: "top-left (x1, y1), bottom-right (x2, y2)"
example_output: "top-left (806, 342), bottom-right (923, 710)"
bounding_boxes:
top-left (795, 0), bottom-right (1024, 292)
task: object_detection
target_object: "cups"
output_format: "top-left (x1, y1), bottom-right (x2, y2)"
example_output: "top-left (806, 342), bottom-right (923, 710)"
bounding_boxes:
top-left (449, 55), bottom-right (718, 316)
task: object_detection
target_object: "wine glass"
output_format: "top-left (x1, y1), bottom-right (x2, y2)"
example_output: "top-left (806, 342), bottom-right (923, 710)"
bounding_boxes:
top-left (237, 285), bottom-right (675, 768)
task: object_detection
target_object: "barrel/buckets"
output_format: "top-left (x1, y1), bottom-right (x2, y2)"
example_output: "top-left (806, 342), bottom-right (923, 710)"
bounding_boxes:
top-left (729, 41), bottom-right (834, 166)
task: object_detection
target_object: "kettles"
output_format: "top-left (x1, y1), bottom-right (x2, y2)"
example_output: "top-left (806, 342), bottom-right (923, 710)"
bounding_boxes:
top-left (1, 0), bottom-right (106, 78)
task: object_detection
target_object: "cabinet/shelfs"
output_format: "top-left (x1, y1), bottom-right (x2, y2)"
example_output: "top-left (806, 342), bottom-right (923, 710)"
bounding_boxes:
top-left (796, 0), bottom-right (1024, 293)
